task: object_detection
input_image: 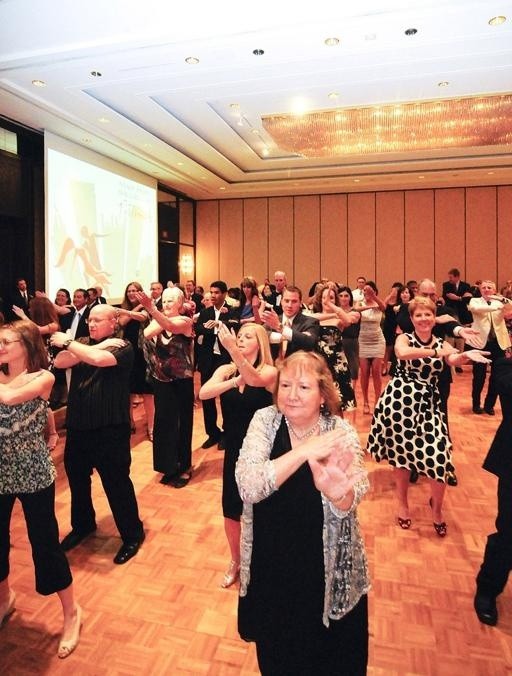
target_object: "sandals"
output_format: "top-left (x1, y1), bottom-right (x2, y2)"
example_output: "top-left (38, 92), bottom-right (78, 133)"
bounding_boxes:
top-left (399, 517), bottom-right (411, 528)
top-left (46, 432), bottom-right (59, 452)
top-left (429, 496), bottom-right (447, 536)
top-left (221, 559), bottom-right (240, 588)
top-left (147, 427), bottom-right (154, 441)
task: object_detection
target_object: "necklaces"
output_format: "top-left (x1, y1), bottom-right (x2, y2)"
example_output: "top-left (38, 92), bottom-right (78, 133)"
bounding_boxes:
top-left (284, 415), bottom-right (320, 443)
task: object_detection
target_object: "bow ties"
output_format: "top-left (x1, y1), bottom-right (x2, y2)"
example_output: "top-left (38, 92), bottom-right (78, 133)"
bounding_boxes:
top-left (275, 291), bottom-right (282, 296)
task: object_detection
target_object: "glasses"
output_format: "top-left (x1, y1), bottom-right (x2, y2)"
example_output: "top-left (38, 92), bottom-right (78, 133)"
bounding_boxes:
top-left (84, 318), bottom-right (112, 324)
top-left (0, 338), bottom-right (22, 345)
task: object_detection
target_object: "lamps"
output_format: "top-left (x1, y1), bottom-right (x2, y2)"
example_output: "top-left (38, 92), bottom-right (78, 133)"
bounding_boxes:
top-left (260, 90), bottom-right (512, 161)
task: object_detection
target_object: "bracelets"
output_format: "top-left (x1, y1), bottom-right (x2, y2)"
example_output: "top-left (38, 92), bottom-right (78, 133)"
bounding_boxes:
top-left (432, 349), bottom-right (439, 359)
top-left (326, 495), bottom-right (344, 504)
top-left (232, 377), bottom-right (238, 388)
top-left (63, 339), bottom-right (73, 350)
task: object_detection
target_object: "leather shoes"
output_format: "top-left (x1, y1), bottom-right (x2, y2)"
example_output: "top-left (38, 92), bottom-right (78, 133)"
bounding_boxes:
top-left (410, 472), bottom-right (419, 482)
top-left (57, 603), bottom-right (81, 658)
top-left (484, 405), bottom-right (494, 414)
top-left (474, 407), bottom-right (482, 412)
top-left (114, 532), bottom-right (145, 564)
top-left (60, 529), bottom-right (85, 551)
top-left (447, 474), bottom-right (458, 485)
top-left (174, 470), bottom-right (192, 488)
top-left (475, 594), bottom-right (498, 625)
top-left (202, 438), bottom-right (217, 448)
top-left (218, 440), bottom-right (226, 449)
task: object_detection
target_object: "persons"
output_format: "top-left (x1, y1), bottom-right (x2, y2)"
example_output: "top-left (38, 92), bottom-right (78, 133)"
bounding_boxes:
top-left (390, 299), bottom-right (492, 537)
top-left (0, 319), bottom-right (88, 659)
top-left (235, 351), bottom-right (363, 676)
top-left (47, 301), bottom-right (150, 565)
top-left (198, 315), bottom-right (286, 587)
top-left (471, 351), bottom-right (512, 626)
top-left (0, 269), bottom-right (511, 489)
top-left (71, 222), bottom-right (113, 295)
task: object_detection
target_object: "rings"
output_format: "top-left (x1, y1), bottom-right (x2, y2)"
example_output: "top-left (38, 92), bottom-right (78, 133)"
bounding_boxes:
top-left (54, 238), bottom-right (115, 299)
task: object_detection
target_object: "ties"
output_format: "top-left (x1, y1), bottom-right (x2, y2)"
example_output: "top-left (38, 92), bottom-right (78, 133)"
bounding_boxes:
top-left (281, 319), bottom-right (289, 358)
